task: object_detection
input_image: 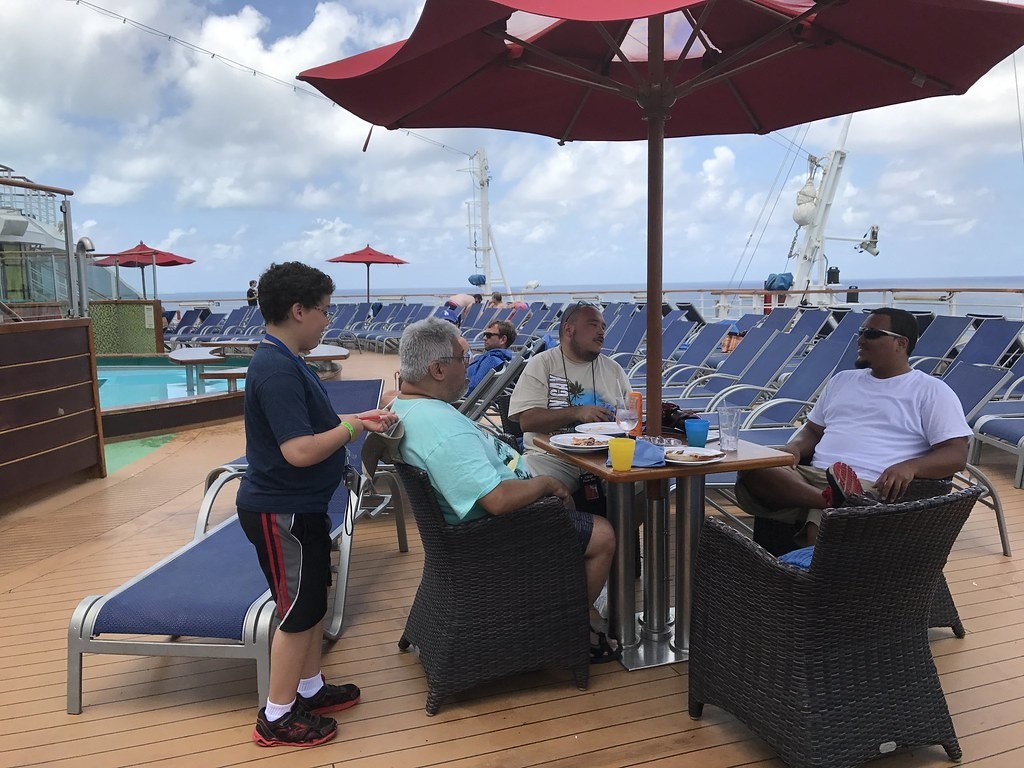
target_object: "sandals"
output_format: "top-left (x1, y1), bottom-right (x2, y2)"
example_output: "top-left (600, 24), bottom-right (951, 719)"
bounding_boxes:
top-left (590, 629), bottom-right (623, 664)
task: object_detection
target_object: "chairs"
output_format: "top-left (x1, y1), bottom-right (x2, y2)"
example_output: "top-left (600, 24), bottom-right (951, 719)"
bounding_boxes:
top-left (67, 378), bottom-right (410, 715)
top-left (396, 457), bottom-right (589, 717)
top-left (160, 300), bottom-right (1024, 580)
top-left (752, 475), bottom-right (967, 640)
top-left (687, 486), bottom-right (989, 768)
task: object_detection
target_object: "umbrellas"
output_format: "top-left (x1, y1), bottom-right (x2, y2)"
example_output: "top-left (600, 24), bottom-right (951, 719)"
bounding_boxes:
top-left (326, 244), bottom-right (407, 304)
top-left (294, 0), bottom-right (1024, 440)
top-left (90, 239), bottom-right (196, 300)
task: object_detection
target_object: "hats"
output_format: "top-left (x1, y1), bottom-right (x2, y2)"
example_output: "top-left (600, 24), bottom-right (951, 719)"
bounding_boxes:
top-left (442, 310), bottom-right (461, 324)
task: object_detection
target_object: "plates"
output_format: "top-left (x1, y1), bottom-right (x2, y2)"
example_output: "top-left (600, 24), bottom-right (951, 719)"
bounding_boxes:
top-left (549, 433), bottom-right (617, 454)
top-left (575, 422), bottom-right (626, 436)
top-left (662, 447), bottom-right (728, 466)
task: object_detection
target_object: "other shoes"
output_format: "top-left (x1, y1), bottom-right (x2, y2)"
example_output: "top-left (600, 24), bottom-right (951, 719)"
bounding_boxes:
top-left (822, 461), bottom-right (863, 507)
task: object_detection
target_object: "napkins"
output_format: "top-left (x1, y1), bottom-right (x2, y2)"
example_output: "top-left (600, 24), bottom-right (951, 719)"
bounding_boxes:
top-left (605, 439), bottom-right (667, 468)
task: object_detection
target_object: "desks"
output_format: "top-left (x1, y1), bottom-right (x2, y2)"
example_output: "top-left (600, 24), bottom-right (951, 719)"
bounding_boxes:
top-left (199, 366), bottom-right (249, 393)
top-left (199, 341), bottom-right (350, 372)
top-left (168, 347), bottom-right (226, 394)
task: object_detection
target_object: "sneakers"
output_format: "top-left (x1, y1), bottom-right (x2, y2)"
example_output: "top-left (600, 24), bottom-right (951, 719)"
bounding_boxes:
top-left (254, 706), bottom-right (337, 747)
top-left (297, 674), bottom-right (361, 714)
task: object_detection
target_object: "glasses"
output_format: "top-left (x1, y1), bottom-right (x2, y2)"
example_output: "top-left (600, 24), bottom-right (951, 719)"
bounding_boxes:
top-left (312, 307), bottom-right (334, 321)
top-left (482, 333), bottom-right (503, 339)
top-left (565, 300), bottom-right (603, 321)
top-left (858, 326), bottom-right (905, 340)
top-left (441, 353), bottom-right (469, 364)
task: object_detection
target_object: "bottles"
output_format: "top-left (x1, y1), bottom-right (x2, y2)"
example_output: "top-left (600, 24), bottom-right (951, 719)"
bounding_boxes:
top-left (628, 392), bottom-right (642, 438)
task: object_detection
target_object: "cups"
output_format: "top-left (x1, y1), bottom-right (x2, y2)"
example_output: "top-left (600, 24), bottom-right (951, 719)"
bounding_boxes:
top-left (608, 438), bottom-right (635, 472)
top-left (684, 419), bottom-right (711, 448)
top-left (718, 407), bottom-right (740, 451)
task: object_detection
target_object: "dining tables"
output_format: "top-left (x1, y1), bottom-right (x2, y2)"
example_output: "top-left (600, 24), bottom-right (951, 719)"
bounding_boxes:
top-left (532, 431), bottom-right (796, 674)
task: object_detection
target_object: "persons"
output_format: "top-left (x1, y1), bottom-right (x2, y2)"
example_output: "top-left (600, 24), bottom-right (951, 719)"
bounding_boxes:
top-left (246, 280), bottom-right (259, 306)
top-left (507, 300), bottom-right (646, 579)
top-left (236, 260), bottom-right (399, 749)
top-left (733, 308), bottom-right (974, 556)
top-left (488, 292), bottom-right (505, 308)
top-left (445, 294), bottom-right (483, 327)
top-left (384, 316), bottom-right (623, 665)
top-left (379, 320), bottom-right (518, 409)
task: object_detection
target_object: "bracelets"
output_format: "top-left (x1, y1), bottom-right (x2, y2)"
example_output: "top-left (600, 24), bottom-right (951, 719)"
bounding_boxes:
top-left (338, 421), bottom-right (355, 443)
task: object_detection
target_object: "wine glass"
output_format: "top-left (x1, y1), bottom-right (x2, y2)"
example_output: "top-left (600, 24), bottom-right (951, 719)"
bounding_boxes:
top-left (616, 397), bottom-right (639, 438)
